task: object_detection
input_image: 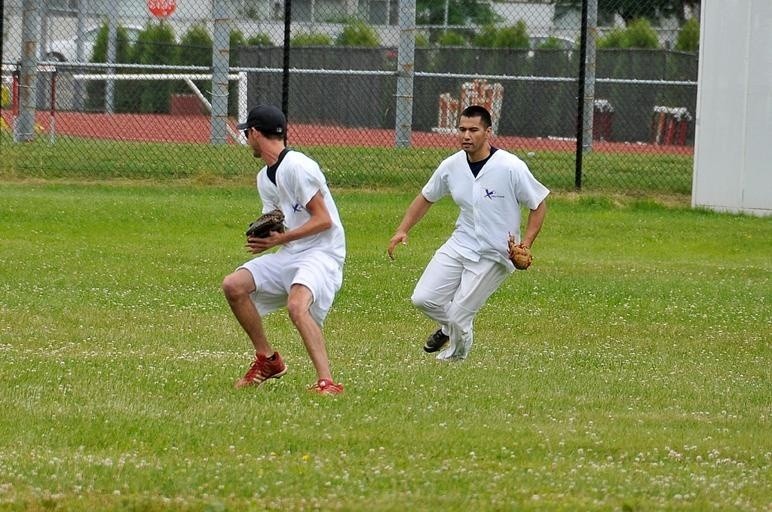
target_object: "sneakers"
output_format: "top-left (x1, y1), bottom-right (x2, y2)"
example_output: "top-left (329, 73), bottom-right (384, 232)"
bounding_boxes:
top-left (310, 379), bottom-right (345, 395)
top-left (423, 329), bottom-right (450, 353)
top-left (233, 353), bottom-right (286, 390)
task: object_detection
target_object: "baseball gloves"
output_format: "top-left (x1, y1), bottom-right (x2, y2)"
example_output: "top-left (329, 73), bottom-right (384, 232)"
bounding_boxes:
top-left (507, 236), bottom-right (532, 270)
top-left (246, 209), bottom-right (285, 243)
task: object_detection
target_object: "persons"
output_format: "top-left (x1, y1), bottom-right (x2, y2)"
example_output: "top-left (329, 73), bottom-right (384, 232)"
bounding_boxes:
top-left (222, 105), bottom-right (345, 397)
top-left (388, 104), bottom-right (551, 362)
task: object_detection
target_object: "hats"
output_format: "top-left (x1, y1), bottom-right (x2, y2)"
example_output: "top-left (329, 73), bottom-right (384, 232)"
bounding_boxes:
top-left (236, 105), bottom-right (286, 136)
